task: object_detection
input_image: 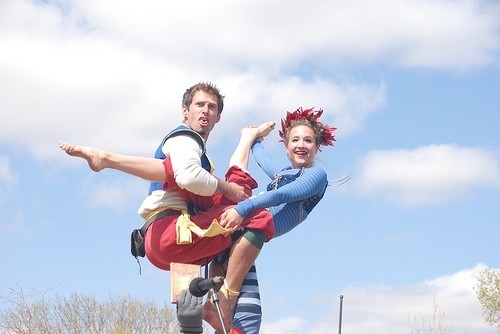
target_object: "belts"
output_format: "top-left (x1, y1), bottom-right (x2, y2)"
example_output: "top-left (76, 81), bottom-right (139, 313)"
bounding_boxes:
top-left (139, 209), bottom-right (183, 236)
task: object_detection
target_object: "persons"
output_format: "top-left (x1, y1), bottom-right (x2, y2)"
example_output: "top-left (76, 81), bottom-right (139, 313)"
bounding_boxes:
top-left (59, 83), bottom-right (337, 334)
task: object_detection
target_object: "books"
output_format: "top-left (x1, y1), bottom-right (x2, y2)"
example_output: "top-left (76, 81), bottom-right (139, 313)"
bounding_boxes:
top-left (170, 262), bottom-right (201, 304)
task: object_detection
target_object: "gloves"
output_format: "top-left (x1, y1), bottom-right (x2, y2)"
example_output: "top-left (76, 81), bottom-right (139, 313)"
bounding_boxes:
top-left (177, 289), bottom-right (205, 334)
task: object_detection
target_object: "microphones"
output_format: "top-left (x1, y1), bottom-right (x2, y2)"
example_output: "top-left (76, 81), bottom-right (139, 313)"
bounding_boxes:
top-left (189, 276), bottom-right (225, 297)
